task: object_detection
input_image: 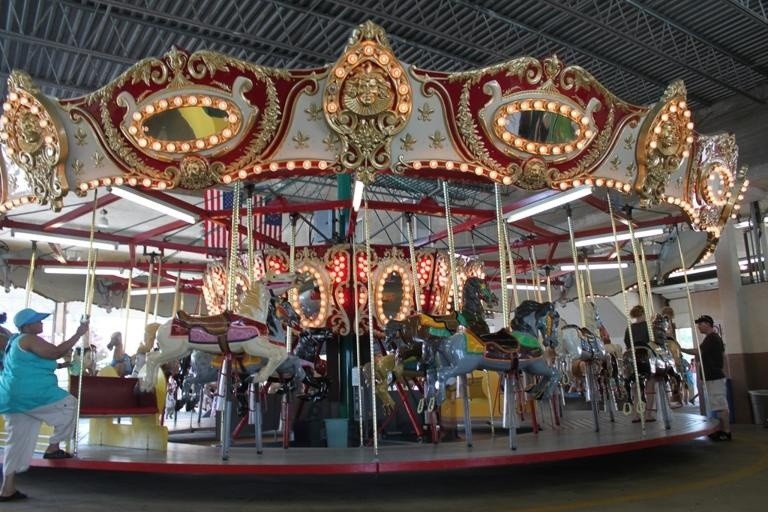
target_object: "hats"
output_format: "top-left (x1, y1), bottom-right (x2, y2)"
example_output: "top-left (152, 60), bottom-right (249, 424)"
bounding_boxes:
top-left (694, 316), bottom-right (714, 324)
top-left (14, 308), bottom-right (52, 329)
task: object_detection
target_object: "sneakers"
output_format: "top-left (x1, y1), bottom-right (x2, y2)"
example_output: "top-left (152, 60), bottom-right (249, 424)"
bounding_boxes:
top-left (707, 430), bottom-right (734, 441)
top-left (632, 417), bottom-right (656, 424)
top-left (43, 449), bottom-right (73, 459)
top-left (0, 490), bottom-right (28, 503)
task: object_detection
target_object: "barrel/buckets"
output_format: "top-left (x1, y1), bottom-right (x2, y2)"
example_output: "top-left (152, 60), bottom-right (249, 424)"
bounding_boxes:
top-left (324, 418), bottom-right (348, 448)
top-left (293, 418), bottom-right (320, 447)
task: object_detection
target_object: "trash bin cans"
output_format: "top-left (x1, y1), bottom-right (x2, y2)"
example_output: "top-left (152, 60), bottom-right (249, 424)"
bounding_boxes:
top-left (705, 378), bottom-right (735, 424)
top-left (747, 390), bottom-right (768, 425)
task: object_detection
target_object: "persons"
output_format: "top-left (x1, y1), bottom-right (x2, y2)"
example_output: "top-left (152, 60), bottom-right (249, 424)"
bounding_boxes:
top-left (0, 309), bottom-right (89, 502)
top-left (625, 306), bottom-right (655, 423)
top-left (679, 315), bottom-right (731, 441)
top-left (676, 353), bottom-right (697, 405)
